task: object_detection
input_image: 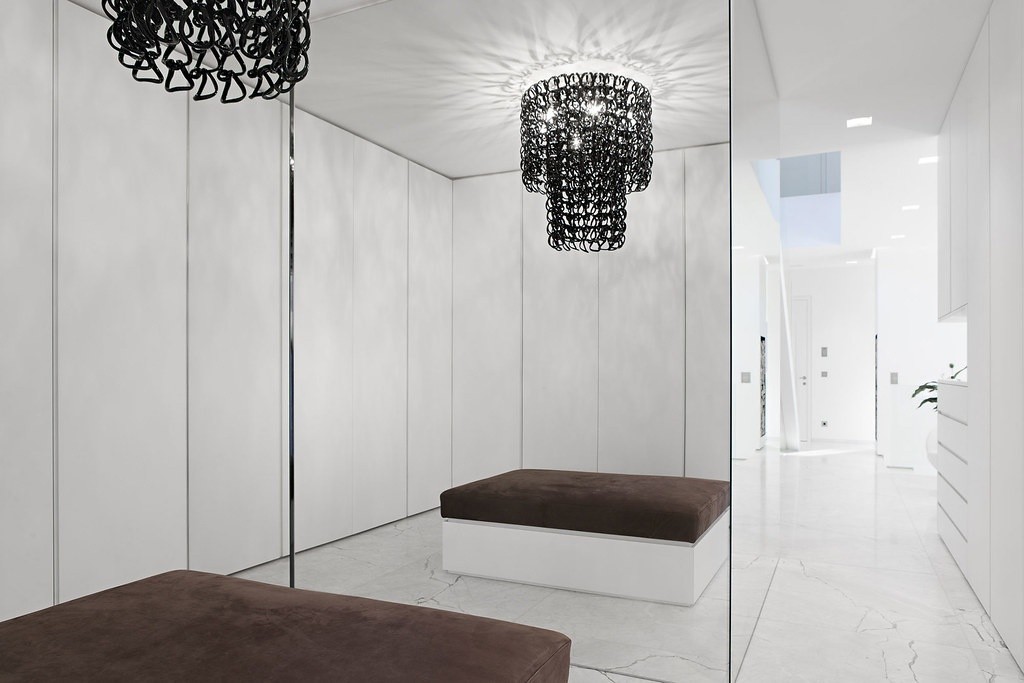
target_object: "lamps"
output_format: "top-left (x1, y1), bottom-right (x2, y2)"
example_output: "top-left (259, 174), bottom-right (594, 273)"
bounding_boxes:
top-left (102, 0), bottom-right (310, 103)
top-left (520, 75), bottom-right (653, 253)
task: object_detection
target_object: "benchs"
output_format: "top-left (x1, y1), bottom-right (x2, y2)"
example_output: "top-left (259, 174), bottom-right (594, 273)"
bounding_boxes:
top-left (0, 570), bottom-right (572, 683)
top-left (442, 468), bottom-right (730, 609)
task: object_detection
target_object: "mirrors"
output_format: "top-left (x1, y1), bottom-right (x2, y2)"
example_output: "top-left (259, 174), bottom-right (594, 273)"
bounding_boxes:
top-left (291, 1), bottom-right (729, 683)
